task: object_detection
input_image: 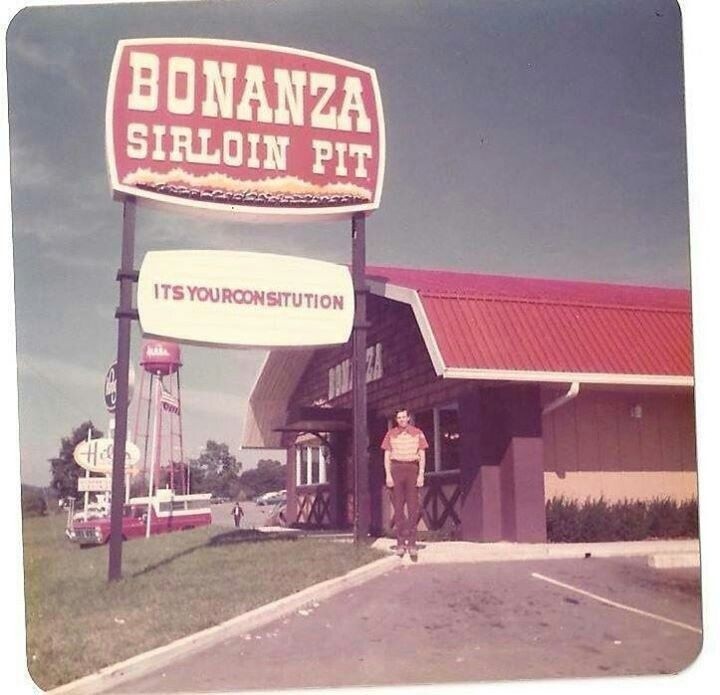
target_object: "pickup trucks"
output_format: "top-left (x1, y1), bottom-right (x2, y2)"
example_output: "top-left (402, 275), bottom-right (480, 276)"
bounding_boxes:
top-left (74, 503), bottom-right (213, 547)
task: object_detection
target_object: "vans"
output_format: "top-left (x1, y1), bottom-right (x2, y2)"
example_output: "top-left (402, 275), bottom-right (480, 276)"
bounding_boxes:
top-left (130, 488), bottom-right (211, 518)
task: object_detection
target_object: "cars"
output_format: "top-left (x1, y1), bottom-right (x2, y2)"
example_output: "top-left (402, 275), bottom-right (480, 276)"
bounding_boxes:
top-left (210, 496), bottom-right (230, 504)
top-left (257, 489), bottom-right (287, 507)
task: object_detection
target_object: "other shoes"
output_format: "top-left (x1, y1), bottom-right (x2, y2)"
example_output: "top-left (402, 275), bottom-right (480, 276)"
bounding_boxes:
top-left (396, 549), bottom-right (404, 556)
top-left (409, 549), bottom-right (418, 558)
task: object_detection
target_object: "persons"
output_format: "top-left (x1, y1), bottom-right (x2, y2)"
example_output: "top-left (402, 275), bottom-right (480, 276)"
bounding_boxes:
top-left (232, 502), bottom-right (245, 526)
top-left (383, 407), bottom-right (429, 556)
